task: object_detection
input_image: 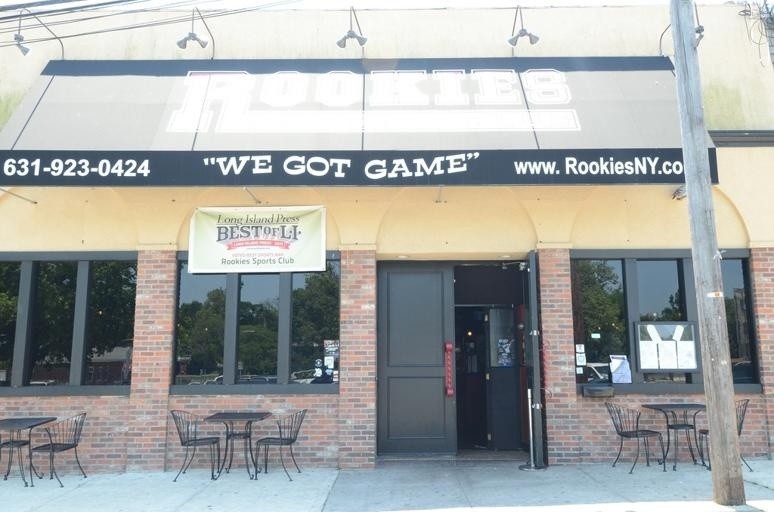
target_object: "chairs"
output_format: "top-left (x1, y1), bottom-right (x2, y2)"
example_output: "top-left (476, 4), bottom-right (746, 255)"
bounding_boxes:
top-left (606, 403), bottom-right (666, 473)
top-left (28, 413), bottom-right (87, 487)
top-left (171, 409), bottom-right (220, 480)
top-left (699, 399), bottom-right (754, 472)
top-left (255, 409), bottom-right (306, 481)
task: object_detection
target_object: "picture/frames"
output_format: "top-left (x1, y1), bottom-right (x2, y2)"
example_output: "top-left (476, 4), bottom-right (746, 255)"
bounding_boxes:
top-left (635, 321), bottom-right (702, 374)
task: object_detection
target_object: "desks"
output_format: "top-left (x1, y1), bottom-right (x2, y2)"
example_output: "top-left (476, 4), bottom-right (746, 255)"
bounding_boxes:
top-left (642, 404), bottom-right (706, 471)
top-left (0, 417), bottom-right (56, 486)
top-left (204, 413), bottom-right (272, 480)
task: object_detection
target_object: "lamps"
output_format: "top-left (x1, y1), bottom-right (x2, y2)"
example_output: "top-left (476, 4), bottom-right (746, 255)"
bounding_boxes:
top-left (336, 7), bottom-right (368, 151)
top-left (659, 16), bottom-right (705, 77)
top-left (7, 8), bottom-right (64, 151)
top-left (178, 8), bottom-right (215, 152)
top-left (508, 5), bottom-right (539, 149)
